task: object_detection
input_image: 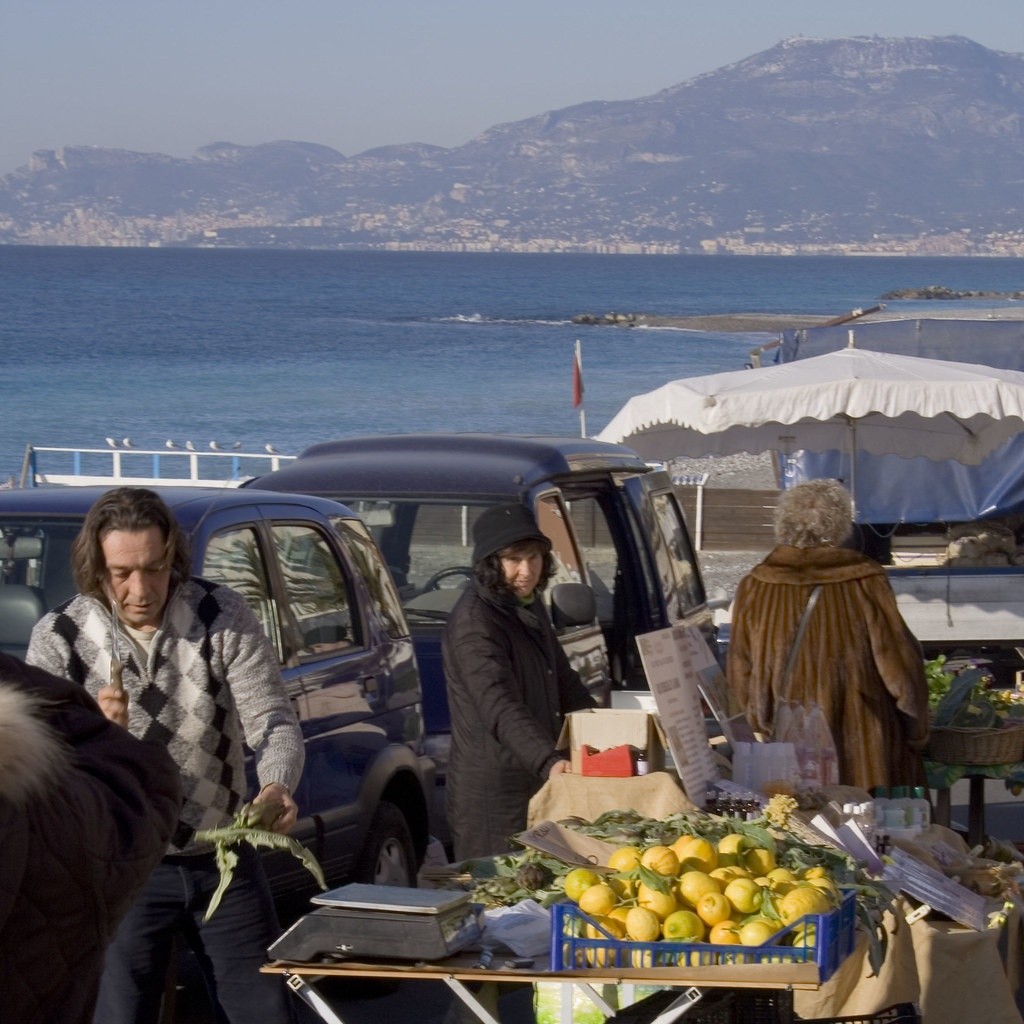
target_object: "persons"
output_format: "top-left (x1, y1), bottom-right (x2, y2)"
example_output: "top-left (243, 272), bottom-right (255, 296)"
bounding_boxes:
top-left (722, 484), bottom-right (932, 794)
top-left (441, 503), bottom-right (599, 1024)
top-left (0, 488), bottom-right (308, 1024)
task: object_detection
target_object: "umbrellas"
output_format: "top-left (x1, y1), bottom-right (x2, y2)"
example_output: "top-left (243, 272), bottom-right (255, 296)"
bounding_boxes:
top-left (571, 355), bottom-right (584, 407)
top-left (577, 331), bottom-right (1023, 530)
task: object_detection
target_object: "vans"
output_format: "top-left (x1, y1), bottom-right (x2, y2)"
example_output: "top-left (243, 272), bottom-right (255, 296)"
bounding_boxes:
top-left (233, 438), bottom-right (710, 870)
top-left (0, 480), bottom-right (436, 1024)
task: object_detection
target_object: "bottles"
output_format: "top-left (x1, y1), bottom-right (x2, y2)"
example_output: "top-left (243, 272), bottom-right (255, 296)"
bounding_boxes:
top-left (636, 751), bottom-right (650, 775)
top-left (776, 700), bottom-right (839, 789)
top-left (731, 741), bottom-right (800, 796)
top-left (843, 785), bottom-right (931, 854)
top-left (702, 790), bottom-right (763, 822)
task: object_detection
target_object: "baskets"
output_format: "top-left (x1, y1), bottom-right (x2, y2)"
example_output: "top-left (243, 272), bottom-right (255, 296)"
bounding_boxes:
top-left (919, 714), bottom-right (1024, 766)
top-left (549, 887), bottom-right (856, 986)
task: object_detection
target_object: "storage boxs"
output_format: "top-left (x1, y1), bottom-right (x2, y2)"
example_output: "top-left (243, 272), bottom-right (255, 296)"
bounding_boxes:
top-left (547, 889), bottom-right (859, 982)
top-left (556, 706), bottom-right (668, 778)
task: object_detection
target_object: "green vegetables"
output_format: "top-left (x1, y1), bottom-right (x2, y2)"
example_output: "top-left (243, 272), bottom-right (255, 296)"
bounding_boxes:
top-left (923, 655), bottom-right (1024, 719)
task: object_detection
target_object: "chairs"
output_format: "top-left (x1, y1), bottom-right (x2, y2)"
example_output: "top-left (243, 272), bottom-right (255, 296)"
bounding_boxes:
top-left (403, 590), bottom-right (465, 620)
top-left (0, 582), bottom-right (46, 647)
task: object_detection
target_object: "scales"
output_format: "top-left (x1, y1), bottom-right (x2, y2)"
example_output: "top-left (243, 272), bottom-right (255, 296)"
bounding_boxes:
top-left (267, 882), bottom-right (481, 961)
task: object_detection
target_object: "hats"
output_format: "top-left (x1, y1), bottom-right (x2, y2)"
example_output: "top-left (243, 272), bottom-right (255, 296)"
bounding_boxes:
top-left (471, 502), bottom-right (552, 567)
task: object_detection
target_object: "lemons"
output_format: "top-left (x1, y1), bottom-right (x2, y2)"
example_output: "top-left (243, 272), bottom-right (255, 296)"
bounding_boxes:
top-left (562, 832), bottom-right (838, 966)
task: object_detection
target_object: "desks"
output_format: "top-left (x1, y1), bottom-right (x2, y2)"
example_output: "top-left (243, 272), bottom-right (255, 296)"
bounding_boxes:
top-left (258, 923), bottom-right (872, 1024)
top-left (526, 766), bottom-right (697, 849)
top-left (920, 757), bottom-right (1024, 859)
